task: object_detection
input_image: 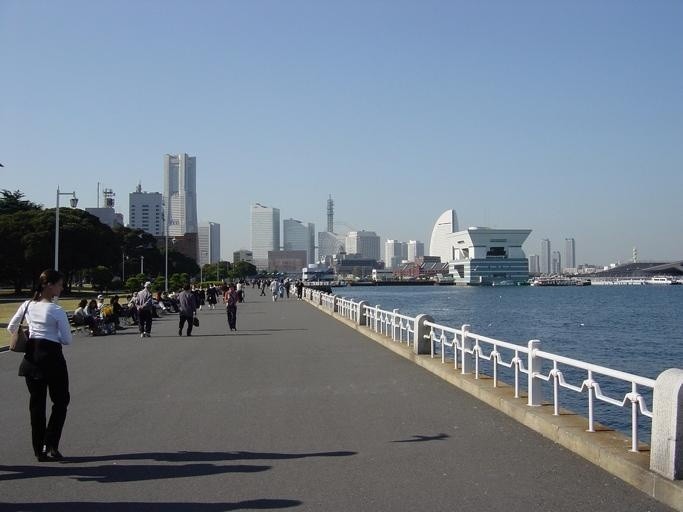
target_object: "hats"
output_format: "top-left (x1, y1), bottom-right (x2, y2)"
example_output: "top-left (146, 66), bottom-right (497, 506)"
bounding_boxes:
top-left (144, 281), bottom-right (152, 288)
top-left (226, 283), bottom-right (237, 289)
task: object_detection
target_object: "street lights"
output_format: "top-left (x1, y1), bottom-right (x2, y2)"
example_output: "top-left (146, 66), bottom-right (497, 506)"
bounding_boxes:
top-left (199, 250), bottom-right (237, 283)
top-left (162, 230), bottom-right (178, 291)
top-left (121, 251), bottom-right (129, 283)
top-left (51, 183), bottom-right (79, 304)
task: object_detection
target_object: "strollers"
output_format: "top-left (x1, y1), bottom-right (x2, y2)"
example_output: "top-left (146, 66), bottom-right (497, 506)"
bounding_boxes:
top-left (89, 302), bottom-right (117, 337)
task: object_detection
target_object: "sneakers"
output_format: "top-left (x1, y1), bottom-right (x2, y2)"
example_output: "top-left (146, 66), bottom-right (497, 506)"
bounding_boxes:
top-left (230, 328), bottom-right (237, 331)
top-left (296, 297), bottom-right (302, 301)
top-left (178, 327), bottom-right (192, 337)
top-left (34, 440), bottom-right (64, 462)
top-left (140, 331), bottom-right (151, 338)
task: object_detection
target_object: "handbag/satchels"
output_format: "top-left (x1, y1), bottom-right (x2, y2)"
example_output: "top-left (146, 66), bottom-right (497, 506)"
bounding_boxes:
top-left (8, 298), bottom-right (34, 353)
top-left (193, 314), bottom-right (200, 327)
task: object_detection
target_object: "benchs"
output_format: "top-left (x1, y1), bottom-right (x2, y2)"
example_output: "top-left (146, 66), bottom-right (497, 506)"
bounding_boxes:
top-left (64, 310), bottom-right (91, 338)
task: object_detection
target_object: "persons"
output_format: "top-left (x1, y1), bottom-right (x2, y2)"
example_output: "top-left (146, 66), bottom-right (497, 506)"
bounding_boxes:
top-left (7, 269), bottom-right (73, 458)
top-left (73, 276), bottom-right (305, 338)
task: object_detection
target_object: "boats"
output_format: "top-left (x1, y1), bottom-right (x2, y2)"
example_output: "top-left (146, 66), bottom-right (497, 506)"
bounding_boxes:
top-left (490, 272), bottom-right (577, 287)
top-left (642, 277), bottom-right (677, 285)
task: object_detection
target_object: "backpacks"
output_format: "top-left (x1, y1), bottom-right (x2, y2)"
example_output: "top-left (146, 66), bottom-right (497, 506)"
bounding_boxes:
top-left (227, 291), bottom-right (236, 313)
